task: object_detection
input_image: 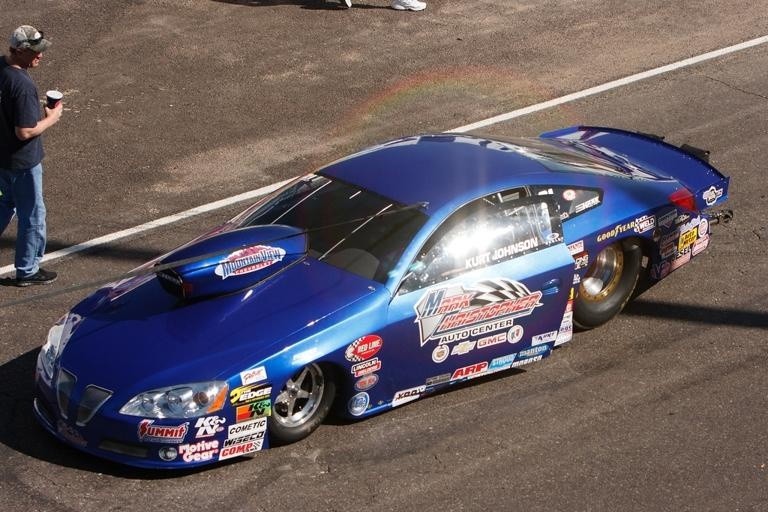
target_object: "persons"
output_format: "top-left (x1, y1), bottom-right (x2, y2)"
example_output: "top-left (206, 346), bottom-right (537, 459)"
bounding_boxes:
top-left (0, 24), bottom-right (66, 287)
top-left (333, 0), bottom-right (427, 11)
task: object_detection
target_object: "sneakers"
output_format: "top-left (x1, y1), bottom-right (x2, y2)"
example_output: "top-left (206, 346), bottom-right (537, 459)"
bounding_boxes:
top-left (390, 0), bottom-right (427, 11)
top-left (17, 268), bottom-right (57, 287)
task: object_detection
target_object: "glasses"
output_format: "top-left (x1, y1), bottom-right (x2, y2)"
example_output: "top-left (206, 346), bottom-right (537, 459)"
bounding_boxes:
top-left (16, 29), bottom-right (43, 46)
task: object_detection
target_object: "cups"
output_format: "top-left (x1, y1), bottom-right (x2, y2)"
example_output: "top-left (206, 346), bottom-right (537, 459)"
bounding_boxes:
top-left (45, 89), bottom-right (64, 108)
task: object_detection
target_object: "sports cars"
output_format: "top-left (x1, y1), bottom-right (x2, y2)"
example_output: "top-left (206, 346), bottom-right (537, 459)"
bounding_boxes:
top-left (34, 126), bottom-right (727, 474)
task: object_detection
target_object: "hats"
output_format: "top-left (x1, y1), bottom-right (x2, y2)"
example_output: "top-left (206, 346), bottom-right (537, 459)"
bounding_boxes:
top-left (11, 26), bottom-right (51, 52)
